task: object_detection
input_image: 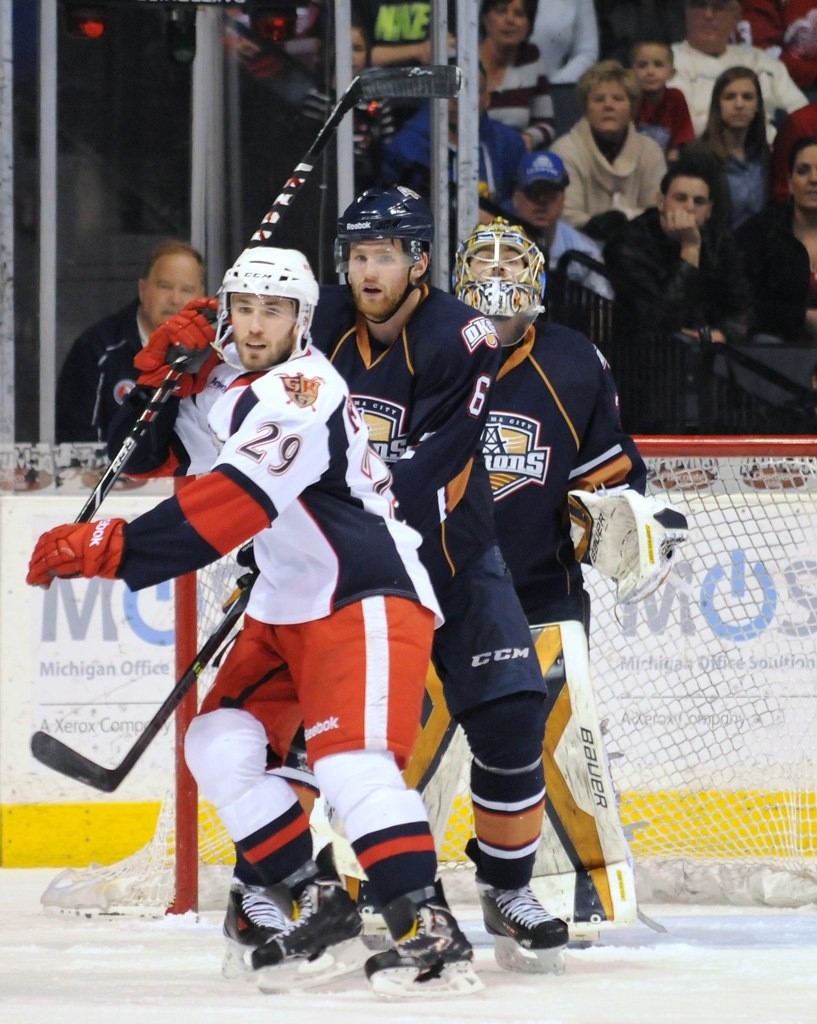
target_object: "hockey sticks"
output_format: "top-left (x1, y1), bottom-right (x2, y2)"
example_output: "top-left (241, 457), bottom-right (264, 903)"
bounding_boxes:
top-left (72, 57), bottom-right (471, 530)
top-left (26, 583), bottom-right (257, 795)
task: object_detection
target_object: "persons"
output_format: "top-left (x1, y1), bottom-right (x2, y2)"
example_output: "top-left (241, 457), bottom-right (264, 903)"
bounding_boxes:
top-left (397, 213), bottom-right (687, 950)
top-left (24, 247), bottom-right (474, 996)
top-left (222, 185), bottom-right (572, 976)
top-left (0, 1), bottom-right (817, 444)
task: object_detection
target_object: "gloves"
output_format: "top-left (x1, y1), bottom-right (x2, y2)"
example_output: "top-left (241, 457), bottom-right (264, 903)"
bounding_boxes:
top-left (26, 518), bottom-right (127, 589)
top-left (134, 299), bottom-right (223, 397)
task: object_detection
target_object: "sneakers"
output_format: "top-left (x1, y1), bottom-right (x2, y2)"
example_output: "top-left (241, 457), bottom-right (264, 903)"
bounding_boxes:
top-left (354, 873), bottom-right (393, 949)
top-left (250, 843), bottom-right (371, 993)
top-left (464, 836), bottom-right (570, 977)
top-left (220, 882), bottom-right (288, 982)
top-left (365, 877), bottom-right (484, 997)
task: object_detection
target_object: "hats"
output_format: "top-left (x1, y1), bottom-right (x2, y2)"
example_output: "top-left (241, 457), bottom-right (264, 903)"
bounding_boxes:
top-left (515, 149), bottom-right (570, 190)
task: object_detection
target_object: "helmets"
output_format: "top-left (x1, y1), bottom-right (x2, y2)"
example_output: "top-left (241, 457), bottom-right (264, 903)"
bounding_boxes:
top-left (452, 216), bottom-right (547, 319)
top-left (214, 244), bottom-right (319, 338)
top-left (332, 183), bottom-right (436, 262)
